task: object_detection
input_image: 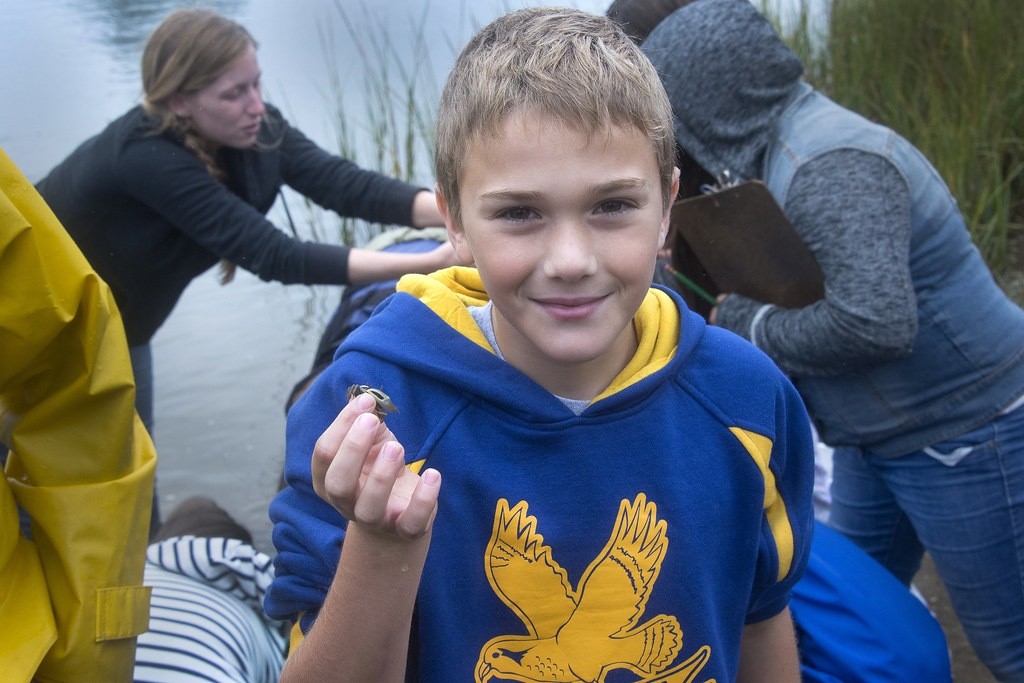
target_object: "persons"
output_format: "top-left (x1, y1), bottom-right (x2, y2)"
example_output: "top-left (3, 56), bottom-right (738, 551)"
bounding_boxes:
top-left (34, 10), bottom-right (473, 541)
top-left (132, 492), bottom-right (286, 683)
top-left (0, 144), bottom-right (155, 682)
top-left (263, 12), bottom-right (815, 683)
top-left (608, 1), bottom-right (1024, 683)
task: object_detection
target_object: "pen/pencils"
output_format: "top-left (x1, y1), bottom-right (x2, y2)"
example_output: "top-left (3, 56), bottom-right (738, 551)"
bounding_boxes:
top-left (665, 263), bottom-right (719, 308)
